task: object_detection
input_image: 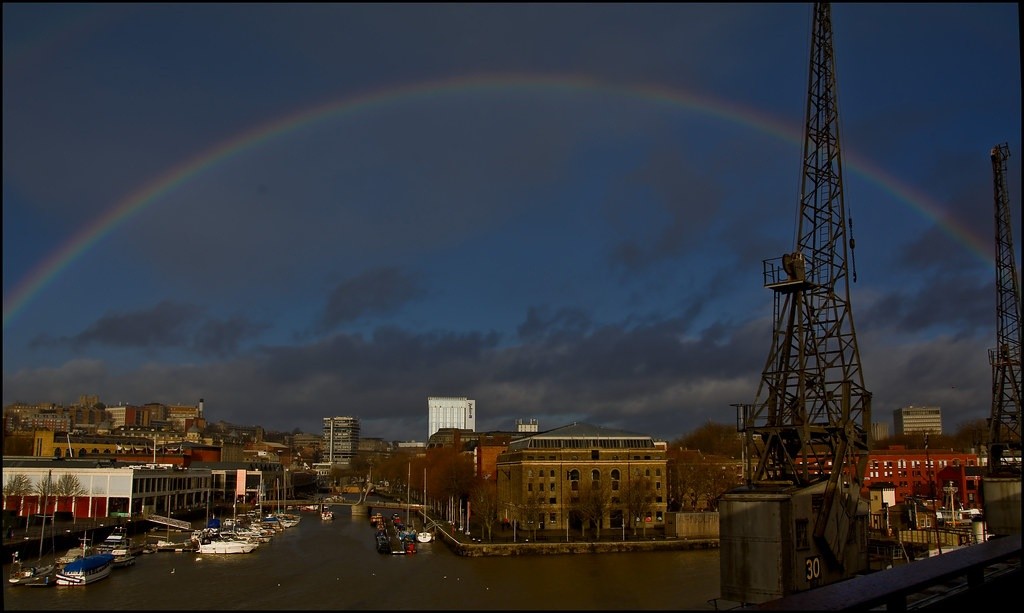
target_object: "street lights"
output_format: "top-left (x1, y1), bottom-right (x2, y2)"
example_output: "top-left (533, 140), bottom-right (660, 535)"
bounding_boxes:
top-left (323, 417), bottom-right (334, 463)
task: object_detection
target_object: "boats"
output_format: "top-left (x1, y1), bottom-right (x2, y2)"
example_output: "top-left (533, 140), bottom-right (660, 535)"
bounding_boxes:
top-left (56, 552), bottom-right (115, 586)
top-left (9, 563), bottom-right (55, 586)
top-left (57, 545), bottom-right (95, 565)
top-left (96, 526), bottom-right (144, 559)
top-left (109, 544), bottom-right (136, 568)
top-left (147, 492), bottom-right (437, 555)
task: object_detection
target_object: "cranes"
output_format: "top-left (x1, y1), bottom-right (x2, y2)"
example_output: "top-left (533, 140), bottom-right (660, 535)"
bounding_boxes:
top-left (717, 0), bottom-right (876, 609)
top-left (984, 143), bottom-right (1024, 484)
